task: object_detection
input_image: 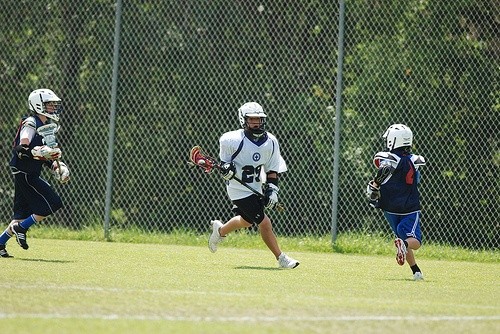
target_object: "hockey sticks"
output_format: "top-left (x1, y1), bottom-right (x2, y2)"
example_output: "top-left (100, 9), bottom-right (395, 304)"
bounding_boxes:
top-left (36, 123), bottom-right (63, 174)
top-left (190, 145), bottom-right (286, 213)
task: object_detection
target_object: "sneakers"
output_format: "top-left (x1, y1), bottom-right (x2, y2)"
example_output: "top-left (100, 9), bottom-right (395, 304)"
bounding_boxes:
top-left (278, 252), bottom-right (300, 269)
top-left (208, 220), bottom-right (223, 253)
top-left (394, 238), bottom-right (406, 266)
top-left (0, 245), bottom-right (9, 257)
top-left (413, 272), bottom-right (424, 280)
top-left (11, 222), bottom-right (29, 249)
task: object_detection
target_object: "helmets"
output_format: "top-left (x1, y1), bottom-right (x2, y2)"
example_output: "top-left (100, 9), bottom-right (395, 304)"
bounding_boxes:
top-left (239, 102), bottom-right (267, 137)
top-left (28, 89), bottom-right (61, 121)
top-left (382, 123), bottom-right (413, 151)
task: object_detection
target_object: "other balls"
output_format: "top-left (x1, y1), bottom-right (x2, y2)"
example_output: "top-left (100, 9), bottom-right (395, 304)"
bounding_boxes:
top-left (198, 159), bottom-right (205, 164)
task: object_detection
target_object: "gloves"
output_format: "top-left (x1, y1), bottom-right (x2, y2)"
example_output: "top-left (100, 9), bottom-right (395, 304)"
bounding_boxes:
top-left (50, 161), bottom-right (69, 183)
top-left (367, 180), bottom-right (380, 212)
top-left (33, 146), bottom-right (62, 160)
top-left (263, 183), bottom-right (280, 211)
top-left (220, 162), bottom-right (237, 180)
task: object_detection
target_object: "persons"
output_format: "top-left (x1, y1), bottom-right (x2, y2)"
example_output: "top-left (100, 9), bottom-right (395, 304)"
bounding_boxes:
top-left (207, 102), bottom-right (300, 269)
top-left (366, 124), bottom-right (426, 280)
top-left (0, 89), bottom-right (69, 258)
